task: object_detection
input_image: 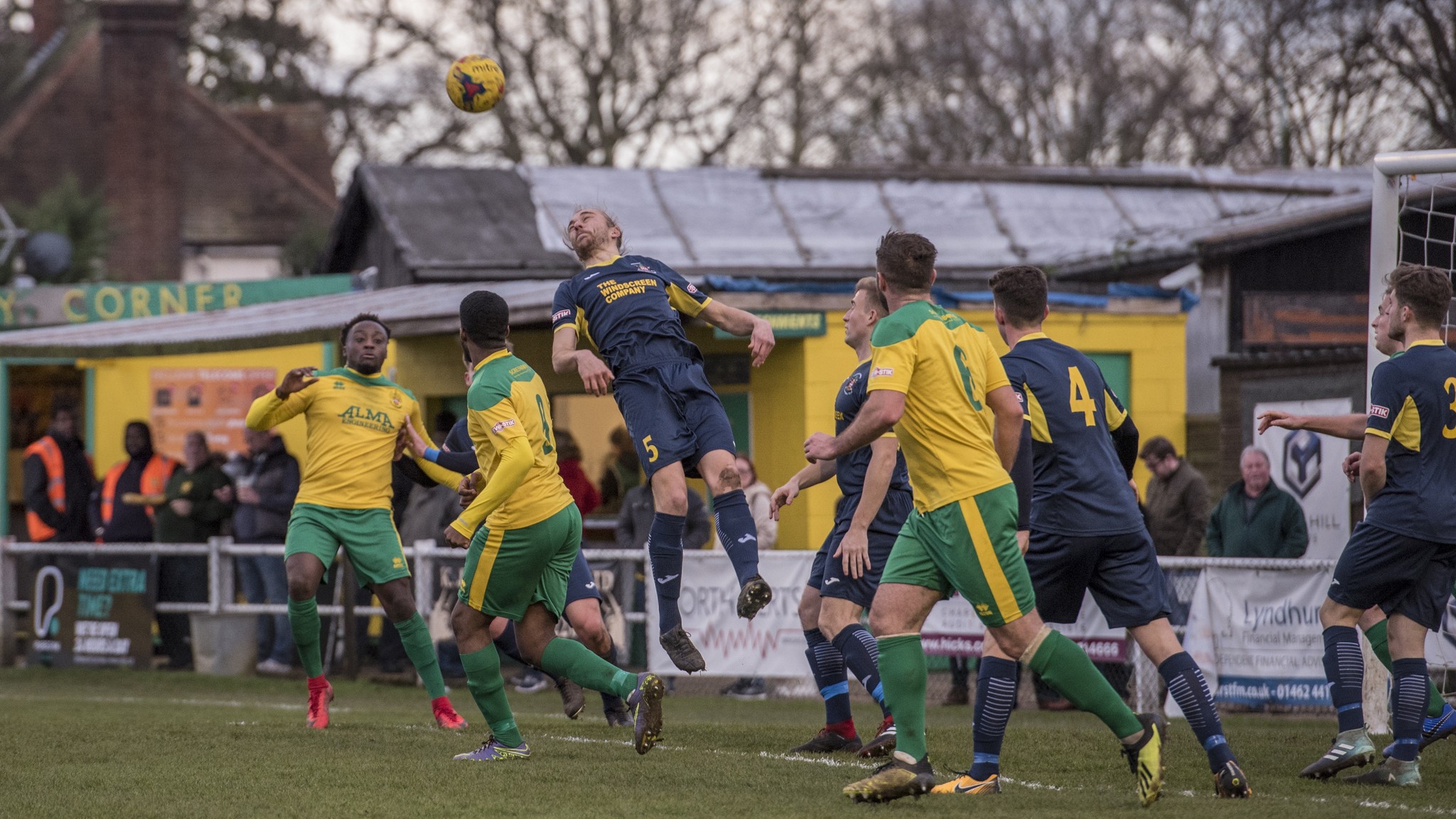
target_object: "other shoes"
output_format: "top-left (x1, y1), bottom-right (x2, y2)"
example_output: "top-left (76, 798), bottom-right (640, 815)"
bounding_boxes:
top-left (509, 671), bottom-right (548, 694)
top-left (255, 658), bottom-right (294, 676)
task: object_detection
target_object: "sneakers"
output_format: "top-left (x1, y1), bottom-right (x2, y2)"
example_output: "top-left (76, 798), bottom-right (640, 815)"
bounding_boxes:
top-left (1298, 722), bottom-right (1376, 781)
top-left (554, 675), bottom-right (586, 720)
top-left (1213, 760), bottom-right (1252, 798)
top-left (306, 680), bottom-right (334, 729)
top-left (930, 763), bottom-right (1002, 795)
top-left (789, 728), bottom-right (864, 754)
top-left (660, 623), bottom-right (705, 675)
top-left (1120, 712), bottom-right (1169, 807)
top-left (434, 705), bottom-right (468, 730)
top-left (1383, 702), bottom-right (1456, 759)
top-left (1342, 755), bottom-right (1421, 787)
top-left (603, 702), bottom-right (635, 728)
top-left (843, 752), bottom-right (934, 803)
top-left (737, 573), bottom-right (771, 620)
top-left (856, 719), bottom-right (926, 758)
top-left (624, 672), bottom-right (665, 754)
top-left (453, 735), bottom-right (530, 761)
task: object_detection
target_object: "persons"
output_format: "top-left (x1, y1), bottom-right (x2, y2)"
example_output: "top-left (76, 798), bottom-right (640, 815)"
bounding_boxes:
top-left (95, 418), bottom-right (187, 543)
top-left (1137, 436), bottom-right (1208, 648)
top-left (551, 210), bottom-right (776, 673)
top-left (352, 416), bottom-right (479, 686)
top-left (715, 452), bottom-right (778, 699)
top-left (439, 291), bottom-right (665, 764)
top-left (1257, 263), bottom-right (1455, 784)
top-left (1205, 444), bottom-right (1312, 556)
top-left (942, 653), bottom-right (1134, 710)
top-left (20, 402), bottom-right (102, 669)
top-left (216, 420), bottom-right (297, 680)
top-left (151, 428), bottom-right (238, 670)
top-left (243, 313), bottom-right (472, 731)
top-left (768, 277), bottom-right (915, 758)
top-left (926, 267), bottom-right (1258, 794)
top-left (803, 229), bottom-right (1174, 808)
top-left (612, 474), bottom-right (712, 688)
top-left (442, 339), bottom-right (636, 729)
top-left (508, 420), bottom-right (642, 694)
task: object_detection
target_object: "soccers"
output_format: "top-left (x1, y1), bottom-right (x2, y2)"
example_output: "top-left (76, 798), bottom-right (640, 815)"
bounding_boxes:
top-left (446, 53), bottom-right (506, 113)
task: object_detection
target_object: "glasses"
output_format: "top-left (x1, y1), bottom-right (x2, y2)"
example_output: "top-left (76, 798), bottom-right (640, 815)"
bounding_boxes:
top-left (1147, 455), bottom-right (1165, 470)
